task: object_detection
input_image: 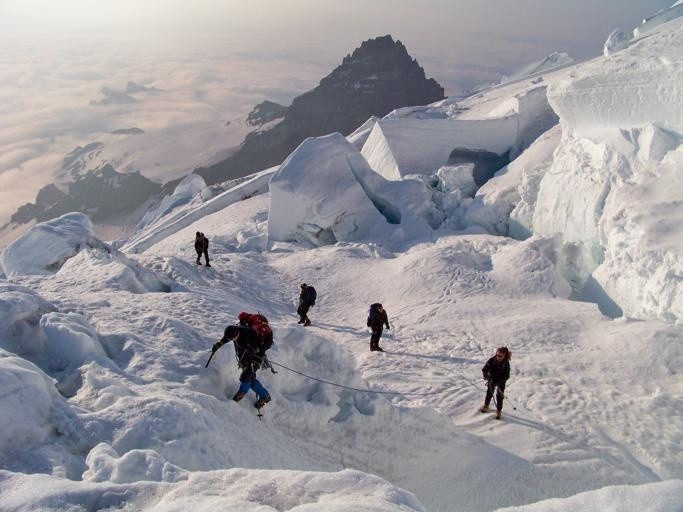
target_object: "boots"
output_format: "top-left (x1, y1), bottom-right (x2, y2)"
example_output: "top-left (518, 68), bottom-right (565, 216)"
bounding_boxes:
top-left (369, 346), bottom-right (383, 352)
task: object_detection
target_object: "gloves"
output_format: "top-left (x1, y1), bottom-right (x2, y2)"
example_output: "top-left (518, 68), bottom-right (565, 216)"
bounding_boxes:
top-left (211, 342), bottom-right (222, 353)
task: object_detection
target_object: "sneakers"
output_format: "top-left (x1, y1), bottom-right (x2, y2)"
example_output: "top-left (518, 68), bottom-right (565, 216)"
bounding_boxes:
top-left (298, 319), bottom-right (311, 326)
top-left (254, 394), bottom-right (272, 409)
top-left (232, 390), bottom-right (246, 403)
top-left (495, 409), bottom-right (502, 420)
top-left (480, 403), bottom-right (490, 414)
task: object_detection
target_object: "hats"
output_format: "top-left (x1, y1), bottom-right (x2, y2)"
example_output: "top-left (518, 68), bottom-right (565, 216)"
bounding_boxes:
top-left (224, 326), bottom-right (239, 338)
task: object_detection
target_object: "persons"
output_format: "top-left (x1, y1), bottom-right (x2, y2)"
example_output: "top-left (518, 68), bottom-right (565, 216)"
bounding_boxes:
top-left (212, 326), bottom-right (271, 408)
top-left (297, 284), bottom-right (316, 326)
top-left (480, 347), bottom-right (510, 419)
top-left (367, 303), bottom-right (389, 351)
top-left (195, 232), bottom-right (211, 267)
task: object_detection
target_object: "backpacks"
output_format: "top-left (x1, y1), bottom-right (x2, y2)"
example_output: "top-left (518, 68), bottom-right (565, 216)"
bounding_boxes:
top-left (237, 311), bottom-right (274, 353)
top-left (366, 302), bottom-right (380, 328)
top-left (302, 285), bottom-right (318, 307)
